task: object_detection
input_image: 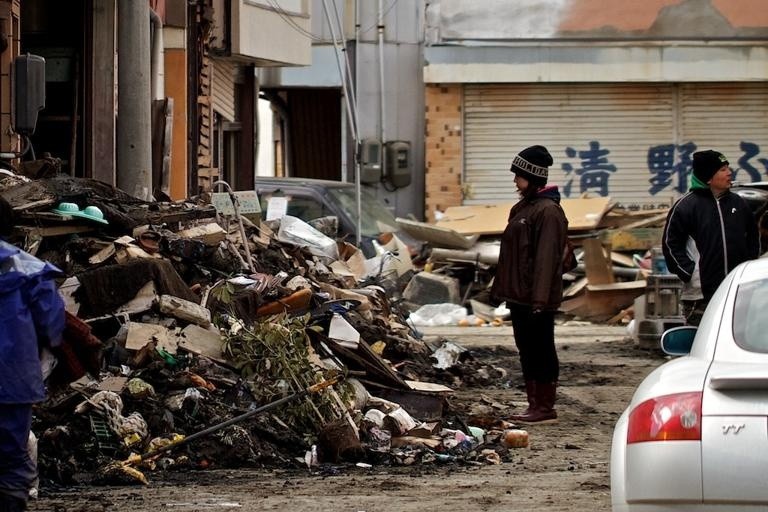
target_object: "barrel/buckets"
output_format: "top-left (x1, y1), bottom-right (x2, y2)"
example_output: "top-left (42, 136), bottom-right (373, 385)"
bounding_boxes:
top-left (650, 247), bottom-right (669, 275)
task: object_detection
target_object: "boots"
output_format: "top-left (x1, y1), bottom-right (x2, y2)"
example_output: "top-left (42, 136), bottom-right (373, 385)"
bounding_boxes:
top-left (511, 379), bottom-right (538, 420)
top-left (517, 380), bottom-right (557, 421)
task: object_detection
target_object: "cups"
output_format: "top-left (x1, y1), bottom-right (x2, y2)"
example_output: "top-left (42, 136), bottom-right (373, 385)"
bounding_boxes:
top-left (652, 255), bottom-right (666, 275)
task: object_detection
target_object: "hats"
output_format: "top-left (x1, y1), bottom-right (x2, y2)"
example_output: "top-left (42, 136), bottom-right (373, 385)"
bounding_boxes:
top-left (509, 144), bottom-right (554, 185)
top-left (692, 149), bottom-right (729, 184)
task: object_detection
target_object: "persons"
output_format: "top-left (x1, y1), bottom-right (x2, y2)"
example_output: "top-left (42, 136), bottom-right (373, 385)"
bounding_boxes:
top-left (0, 194), bottom-right (88, 511)
top-left (489, 144), bottom-right (569, 408)
top-left (661, 150), bottom-right (754, 327)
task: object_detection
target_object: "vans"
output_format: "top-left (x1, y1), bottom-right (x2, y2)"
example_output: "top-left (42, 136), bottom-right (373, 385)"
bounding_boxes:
top-left (255, 176), bottom-right (430, 268)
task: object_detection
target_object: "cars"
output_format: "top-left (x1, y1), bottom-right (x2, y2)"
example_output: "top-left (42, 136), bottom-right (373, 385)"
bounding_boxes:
top-left (609, 252), bottom-right (768, 512)
